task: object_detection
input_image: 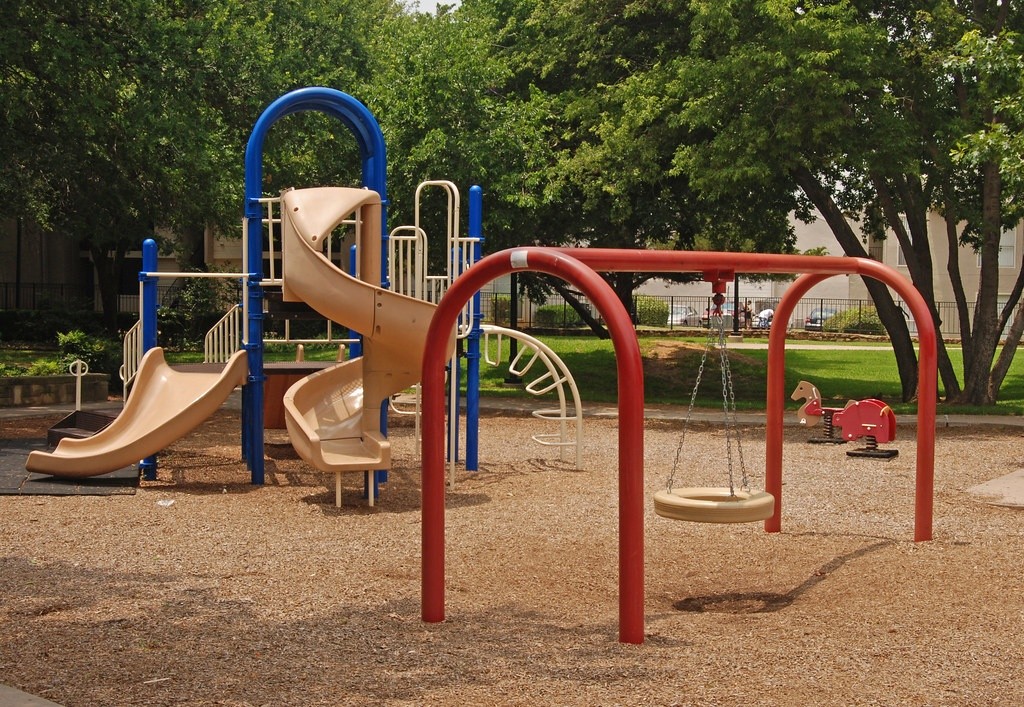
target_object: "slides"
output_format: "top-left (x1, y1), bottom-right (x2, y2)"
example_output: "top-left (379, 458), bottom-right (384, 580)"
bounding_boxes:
top-left (25, 345), bottom-right (248, 475)
top-left (279, 185), bottom-right (457, 471)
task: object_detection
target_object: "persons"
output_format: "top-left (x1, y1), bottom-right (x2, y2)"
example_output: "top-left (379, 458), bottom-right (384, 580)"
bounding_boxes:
top-left (745, 301), bottom-right (753, 330)
top-left (757, 309), bottom-right (775, 328)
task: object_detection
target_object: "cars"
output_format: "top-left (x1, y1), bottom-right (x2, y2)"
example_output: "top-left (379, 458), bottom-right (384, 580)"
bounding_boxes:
top-left (805, 307), bottom-right (838, 331)
top-left (700, 299), bottom-right (752, 329)
top-left (667, 304), bottom-right (704, 328)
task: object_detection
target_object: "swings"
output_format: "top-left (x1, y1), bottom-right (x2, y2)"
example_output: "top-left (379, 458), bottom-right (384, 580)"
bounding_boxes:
top-left (652, 312), bottom-right (776, 524)
